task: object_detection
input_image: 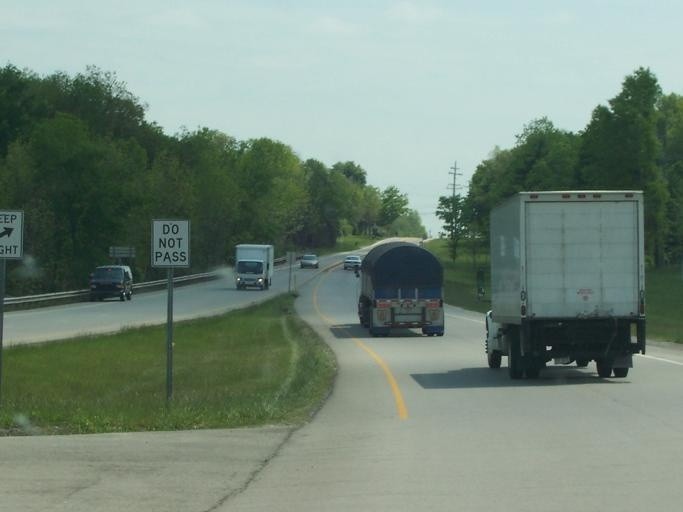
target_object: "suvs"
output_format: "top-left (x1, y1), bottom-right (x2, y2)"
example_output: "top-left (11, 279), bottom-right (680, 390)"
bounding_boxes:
top-left (90, 264), bottom-right (134, 301)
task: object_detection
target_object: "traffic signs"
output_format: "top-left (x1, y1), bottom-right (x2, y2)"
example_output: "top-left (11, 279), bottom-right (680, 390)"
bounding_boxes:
top-left (0, 210), bottom-right (23, 258)
top-left (152, 219), bottom-right (190, 268)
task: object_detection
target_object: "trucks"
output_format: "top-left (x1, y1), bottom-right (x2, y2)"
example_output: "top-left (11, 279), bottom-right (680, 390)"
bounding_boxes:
top-left (353, 242), bottom-right (445, 336)
top-left (235, 245), bottom-right (275, 290)
top-left (485, 191), bottom-right (647, 375)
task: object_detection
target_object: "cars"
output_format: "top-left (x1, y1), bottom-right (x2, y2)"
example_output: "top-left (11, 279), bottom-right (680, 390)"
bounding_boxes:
top-left (343, 256), bottom-right (362, 268)
top-left (300, 255), bottom-right (319, 268)
